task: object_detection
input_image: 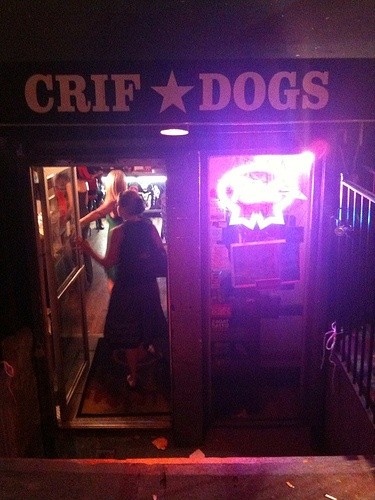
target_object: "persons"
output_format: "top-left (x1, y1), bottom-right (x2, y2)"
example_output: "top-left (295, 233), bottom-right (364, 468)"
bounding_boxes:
top-left (75, 165), bottom-right (166, 239)
top-left (53, 176), bottom-right (75, 267)
top-left (77, 190), bottom-right (173, 397)
top-left (78, 166), bottom-right (133, 298)
top-left (55, 173), bottom-right (79, 269)
